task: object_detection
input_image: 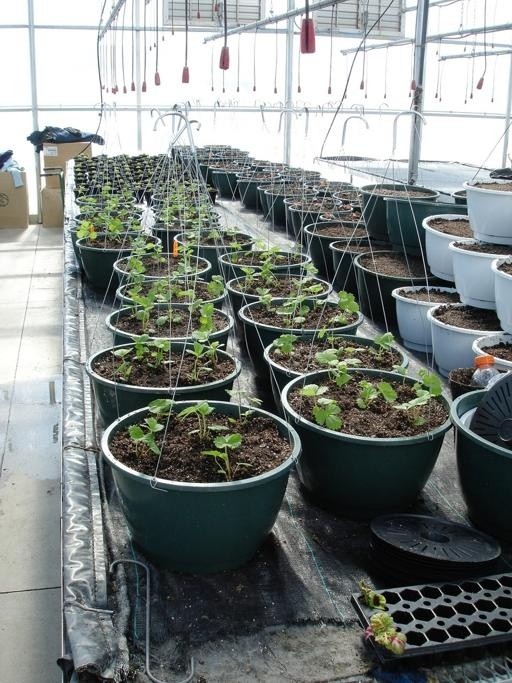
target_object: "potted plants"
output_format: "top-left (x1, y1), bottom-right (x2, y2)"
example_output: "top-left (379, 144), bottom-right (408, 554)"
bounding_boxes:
top-left (71, 153), bottom-right (225, 228)
top-left (277, 365), bottom-right (449, 511)
top-left (110, 277), bottom-right (229, 311)
top-left (114, 249), bottom-right (214, 282)
top-left (236, 296), bottom-right (366, 390)
top-left (97, 394), bottom-right (305, 563)
top-left (171, 229), bottom-right (256, 280)
top-left (100, 302), bottom-right (238, 354)
top-left (225, 271), bottom-right (336, 351)
top-left (81, 339), bottom-right (245, 427)
top-left (259, 331), bottom-right (412, 423)
top-left (217, 248), bottom-right (316, 277)
top-left (74, 231), bottom-right (164, 296)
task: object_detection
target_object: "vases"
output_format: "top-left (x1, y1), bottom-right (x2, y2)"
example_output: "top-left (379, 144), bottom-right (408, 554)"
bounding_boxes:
top-left (359, 180), bottom-right (441, 248)
top-left (445, 385), bottom-right (512, 540)
top-left (391, 284), bottom-right (465, 353)
top-left (468, 332), bottom-right (511, 382)
top-left (380, 194), bottom-right (470, 257)
top-left (449, 238), bottom-right (510, 312)
top-left (175, 142), bottom-right (371, 244)
top-left (303, 219), bottom-right (368, 282)
top-left (489, 256), bottom-right (512, 332)
top-left (460, 177), bottom-right (512, 246)
top-left (353, 249), bottom-right (435, 331)
top-left (327, 240), bottom-right (392, 296)
top-left (451, 366), bottom-right (508, 401)
top-left (424, 301), bottom-right (501, 387)
top-left (420, 211), bottom-right (472, 285)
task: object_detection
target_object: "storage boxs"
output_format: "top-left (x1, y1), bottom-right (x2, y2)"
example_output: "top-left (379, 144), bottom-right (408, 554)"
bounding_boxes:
top-left (43, 140), bottom-right (92, 187)
top-left (41, 187), bottom-right (66, 227)
top-left (1, 167), bottom-right (29, 229)
